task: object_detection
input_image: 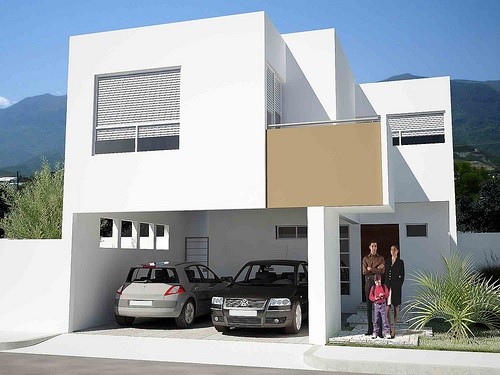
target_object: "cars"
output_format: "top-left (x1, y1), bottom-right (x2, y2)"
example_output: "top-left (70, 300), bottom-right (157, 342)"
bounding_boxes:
top-left (113, 261), bottom-right (230, 329)
top-left (210, 259), bottom-right (308, 334)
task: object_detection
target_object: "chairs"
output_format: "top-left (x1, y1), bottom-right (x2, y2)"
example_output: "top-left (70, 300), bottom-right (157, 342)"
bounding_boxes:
top-left (282, 272), bottom-right (294, 280)
top-left (256, 272), bottom-right (263, 279)
top-left (148, 269), bottom-right (195, 281)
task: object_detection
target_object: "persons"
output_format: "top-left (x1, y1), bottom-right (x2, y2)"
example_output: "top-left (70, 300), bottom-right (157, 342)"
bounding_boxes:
top-left (362, 240), bottom-right (386, 335)
top-left (369, 272), bottom-right (392, 339)
top-left (381, 244), bottom-right (405, 338)
